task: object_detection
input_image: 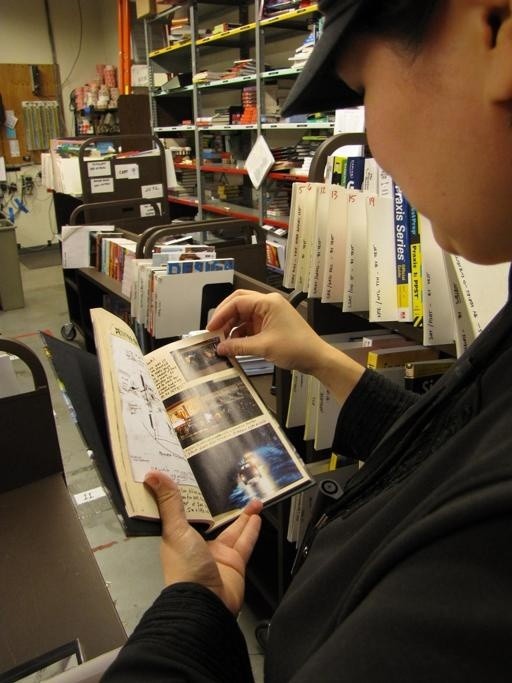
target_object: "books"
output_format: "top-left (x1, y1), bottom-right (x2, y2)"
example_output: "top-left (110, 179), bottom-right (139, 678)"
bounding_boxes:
top-left (31, 1), bottom-right (512, 552)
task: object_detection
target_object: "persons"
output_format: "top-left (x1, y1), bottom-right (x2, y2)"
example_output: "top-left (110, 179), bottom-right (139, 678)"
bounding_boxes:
top-left (97, 1), bottom-right (512, 681)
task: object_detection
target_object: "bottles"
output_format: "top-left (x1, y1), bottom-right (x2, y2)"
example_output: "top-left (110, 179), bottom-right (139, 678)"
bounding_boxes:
top-left (75, 63), bottom-right (121, 113)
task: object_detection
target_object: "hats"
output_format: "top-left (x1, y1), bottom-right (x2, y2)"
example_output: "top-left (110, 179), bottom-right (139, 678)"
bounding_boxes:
top-left (279, 0), bottom-right (362, 118)
